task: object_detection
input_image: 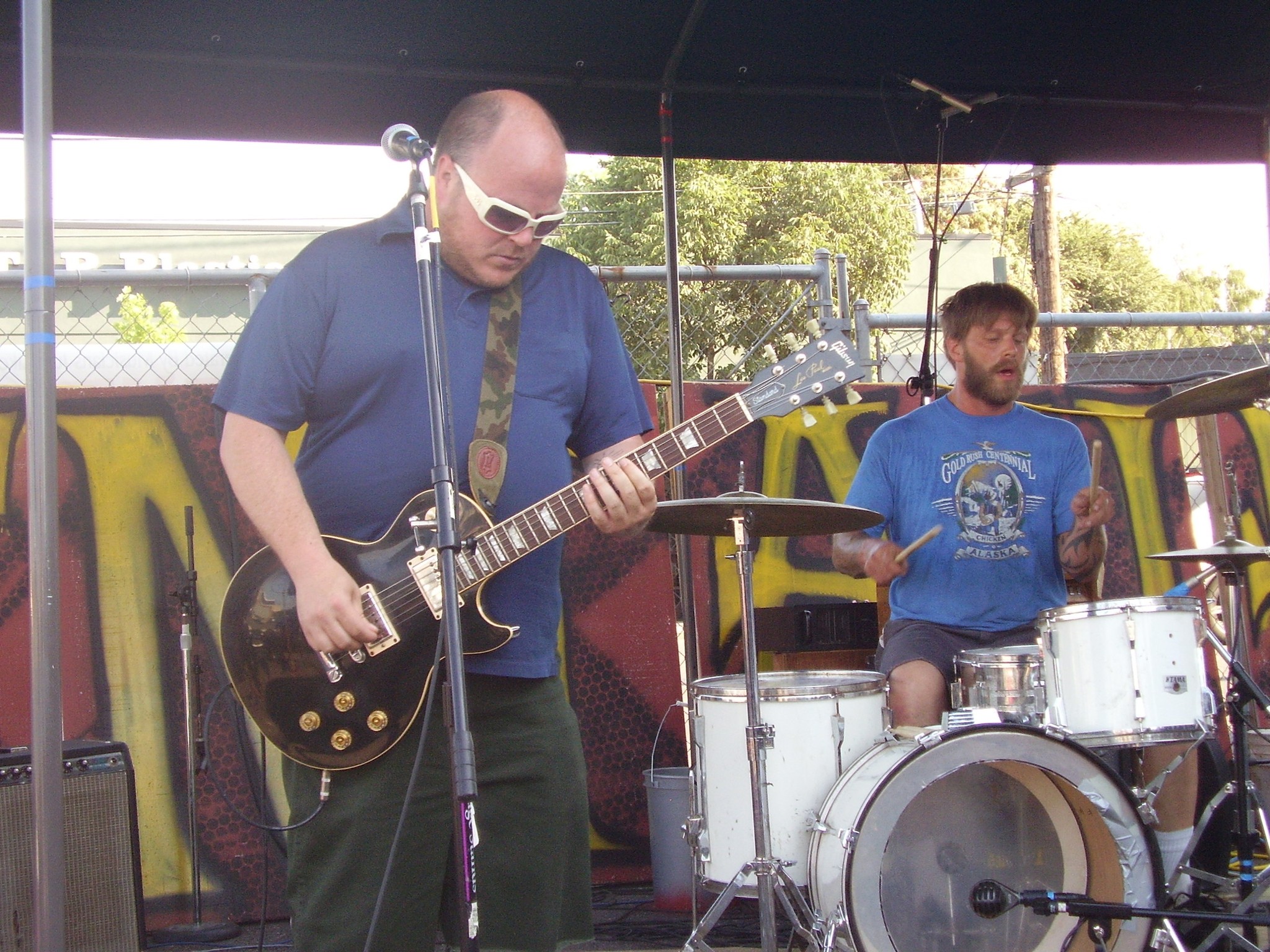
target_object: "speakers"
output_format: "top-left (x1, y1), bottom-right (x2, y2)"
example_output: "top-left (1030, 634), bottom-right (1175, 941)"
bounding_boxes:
top-left (0, 738), bottom-right (148, 952)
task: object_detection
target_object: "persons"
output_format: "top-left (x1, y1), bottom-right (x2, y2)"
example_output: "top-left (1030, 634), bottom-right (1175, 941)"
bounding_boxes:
top-left (832, 283), bottom-right (1257, 952)
top-left (211, 88), bottom-right (659, 952)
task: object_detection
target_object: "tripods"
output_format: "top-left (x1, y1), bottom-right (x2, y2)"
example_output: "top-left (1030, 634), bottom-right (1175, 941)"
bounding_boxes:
top-left (1162, 562), bottom-right (1270, 902)
top-left (681, 507), bottom-right (823, 952)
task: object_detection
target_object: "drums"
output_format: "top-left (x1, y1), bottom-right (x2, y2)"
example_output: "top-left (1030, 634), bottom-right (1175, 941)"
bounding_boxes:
top-left (1036, 593), bottom-right (1219, 753)
top-left (955, 644), bottom-right (1045, 730)
top-left (805, 719), bottom-right (1166, 951)
top-left (686, 668), bottom-right (892, 900)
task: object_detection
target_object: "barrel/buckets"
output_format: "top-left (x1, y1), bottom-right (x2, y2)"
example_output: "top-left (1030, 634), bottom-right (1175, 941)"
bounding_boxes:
top-left (643, 701), bottom-right (736, 910)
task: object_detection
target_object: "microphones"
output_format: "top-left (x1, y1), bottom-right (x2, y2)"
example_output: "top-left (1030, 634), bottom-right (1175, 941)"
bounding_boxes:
top-left (380, 124), bottom-right (432, 161)
top-left (969, 880), bottom-right (1088, 918)
top-left (1165, 559), bottom-right (1227, 597)
top-left (892, 70), bottom-right (972, 114)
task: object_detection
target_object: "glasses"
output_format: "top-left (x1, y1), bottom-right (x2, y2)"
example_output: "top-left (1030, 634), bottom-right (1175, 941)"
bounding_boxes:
top-left (452, 161), bottom-right (567, 239)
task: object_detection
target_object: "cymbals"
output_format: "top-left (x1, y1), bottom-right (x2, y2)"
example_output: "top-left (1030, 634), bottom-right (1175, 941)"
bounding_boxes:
top-left (1144, 538), bottom-right (1270, 570)
top-left (644, 488), bottom-right (888, 541)
top-left (1142, 364), bottom-right (1269, 420)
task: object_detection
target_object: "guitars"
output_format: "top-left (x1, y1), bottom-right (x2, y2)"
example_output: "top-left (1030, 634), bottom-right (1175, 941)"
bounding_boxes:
top-left (217, 314), bottom-right (872, 775)
